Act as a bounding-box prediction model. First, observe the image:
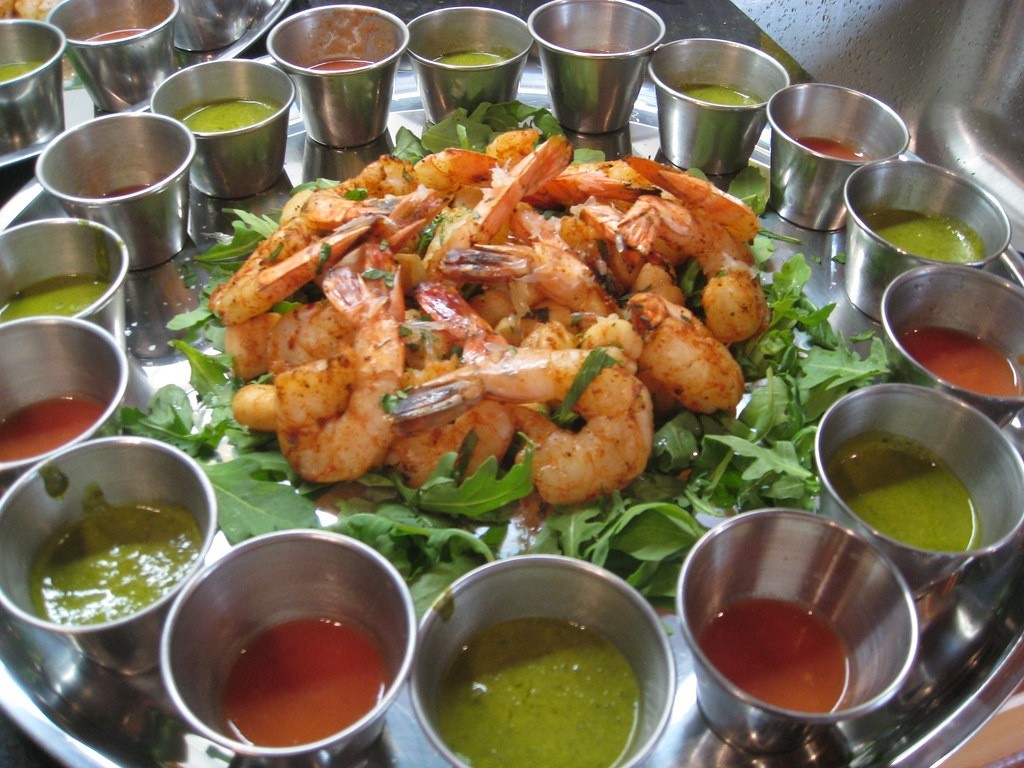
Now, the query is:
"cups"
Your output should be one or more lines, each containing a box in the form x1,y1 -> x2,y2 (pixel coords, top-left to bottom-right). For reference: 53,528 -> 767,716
816,381 -> 1024,599
528,0 -> 666,133
161,530 -> 417,768
409,554 -> 677,768
151,58 -> 298,198
766,82 -> 912,231
266,3 -> 410,148
843,161 -> 1012,321
677,508 -> 918,756
879,264 -> 1024,426
176,0 -> 249,52
406,6 -> 533,124
0,1 -> 217,768
647,38 -> 790,175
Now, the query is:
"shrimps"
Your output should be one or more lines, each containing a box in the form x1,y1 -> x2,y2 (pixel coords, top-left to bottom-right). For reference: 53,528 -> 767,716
202,128 -> 767,509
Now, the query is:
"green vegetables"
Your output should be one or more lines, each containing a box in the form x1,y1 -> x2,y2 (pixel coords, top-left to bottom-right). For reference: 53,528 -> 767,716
118,101 -> 894,636
